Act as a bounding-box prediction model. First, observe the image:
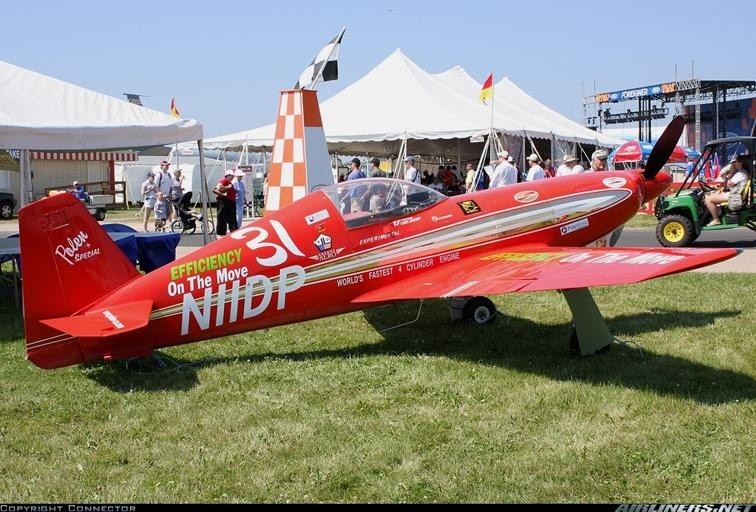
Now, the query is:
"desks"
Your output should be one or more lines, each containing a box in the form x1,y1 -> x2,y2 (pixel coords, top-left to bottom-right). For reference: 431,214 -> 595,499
0,230 -> 181,311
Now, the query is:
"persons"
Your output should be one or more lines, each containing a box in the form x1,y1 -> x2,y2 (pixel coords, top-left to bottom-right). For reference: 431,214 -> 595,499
335,150 -> 608,212
67,181 -> 85,200
705,155 -> 750,226
141,161 -> 268,240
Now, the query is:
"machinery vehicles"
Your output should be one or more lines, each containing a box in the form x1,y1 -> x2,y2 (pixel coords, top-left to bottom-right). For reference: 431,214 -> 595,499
68,189 -> 116,222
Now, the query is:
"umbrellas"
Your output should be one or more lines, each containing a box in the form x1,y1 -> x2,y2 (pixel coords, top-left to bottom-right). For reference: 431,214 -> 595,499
608,140 -> 702,174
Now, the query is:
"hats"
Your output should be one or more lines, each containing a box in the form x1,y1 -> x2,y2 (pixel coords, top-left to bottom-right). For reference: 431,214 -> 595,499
368,157 -> 380,164
161,161 -> 170,166
566,156 -> 577,161
497,151 -> 509,158
234,170 -> 246,176
402,156 -> 415,163
592,150 -> 607,159
225,170 -> 233,176
351,158 -> 360,164
148,172 -> 156,177
527,154 -> 539,161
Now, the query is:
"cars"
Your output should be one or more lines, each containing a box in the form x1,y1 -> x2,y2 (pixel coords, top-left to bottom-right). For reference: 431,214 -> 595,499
0,192 -> 16,218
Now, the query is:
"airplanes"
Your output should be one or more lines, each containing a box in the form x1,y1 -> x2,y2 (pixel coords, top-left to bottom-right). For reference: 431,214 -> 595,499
16,77 -> 747,369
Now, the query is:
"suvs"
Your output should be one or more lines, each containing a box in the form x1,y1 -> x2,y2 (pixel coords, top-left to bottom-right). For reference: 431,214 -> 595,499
654,136 -> 756,245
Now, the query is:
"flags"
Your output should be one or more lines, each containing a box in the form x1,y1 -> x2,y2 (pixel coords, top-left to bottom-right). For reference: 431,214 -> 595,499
171,97 -> 179,117
479,74 -> 493,102
294,27 -> 345,90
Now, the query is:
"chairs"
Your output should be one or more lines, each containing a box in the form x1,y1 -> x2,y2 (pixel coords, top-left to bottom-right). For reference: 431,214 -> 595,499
719,178 -> 751,207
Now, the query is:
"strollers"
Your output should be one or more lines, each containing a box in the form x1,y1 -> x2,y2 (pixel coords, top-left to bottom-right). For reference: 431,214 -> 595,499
145,186 -> 215,237
